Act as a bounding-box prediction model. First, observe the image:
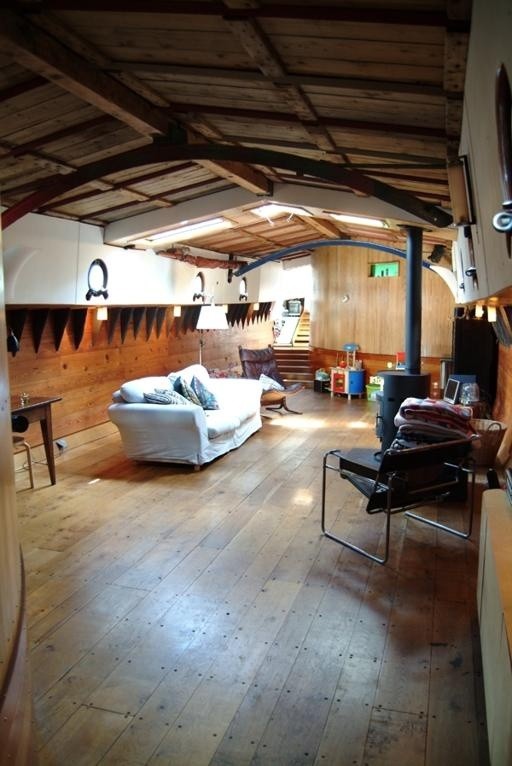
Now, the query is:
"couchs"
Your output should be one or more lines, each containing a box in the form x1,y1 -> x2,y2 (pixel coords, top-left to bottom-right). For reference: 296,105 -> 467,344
107,363 -> 265,472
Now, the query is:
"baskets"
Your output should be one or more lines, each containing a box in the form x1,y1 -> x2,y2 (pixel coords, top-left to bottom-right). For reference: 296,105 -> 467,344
470,418 -> 508,465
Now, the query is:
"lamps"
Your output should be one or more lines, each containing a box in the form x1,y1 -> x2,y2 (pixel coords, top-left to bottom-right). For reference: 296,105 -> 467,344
173,305 -> 181,317
446,154 -> 478,229
487,306 -> 497,323
194,238 -> 459,331
96,306 -> 108,321
474,304 -> 484,317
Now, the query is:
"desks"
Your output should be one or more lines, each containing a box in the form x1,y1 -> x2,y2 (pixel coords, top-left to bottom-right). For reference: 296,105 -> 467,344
11,395 -> 62,486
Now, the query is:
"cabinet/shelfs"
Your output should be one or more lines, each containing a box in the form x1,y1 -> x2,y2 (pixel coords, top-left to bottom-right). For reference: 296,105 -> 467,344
328,366 -> 365,400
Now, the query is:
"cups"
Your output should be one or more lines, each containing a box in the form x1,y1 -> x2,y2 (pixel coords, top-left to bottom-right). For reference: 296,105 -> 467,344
20,392 -> 29,404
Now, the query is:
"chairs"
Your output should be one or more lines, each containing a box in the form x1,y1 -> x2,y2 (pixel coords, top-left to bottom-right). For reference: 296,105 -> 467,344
238,344 -> 305,415
11,415 -> 34,489
321,434 -> 482,564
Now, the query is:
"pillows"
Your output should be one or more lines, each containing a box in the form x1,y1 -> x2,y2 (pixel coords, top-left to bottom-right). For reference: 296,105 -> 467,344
154,388 -> 192,405
190,376 -> 220,410
143,393 -> 179,405
167,364 -> 209,386
173,376 -> 201,405
119,376 -> 174,402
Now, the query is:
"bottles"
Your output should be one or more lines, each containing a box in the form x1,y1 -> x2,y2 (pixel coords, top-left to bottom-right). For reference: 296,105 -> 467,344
432,383 -> 439,398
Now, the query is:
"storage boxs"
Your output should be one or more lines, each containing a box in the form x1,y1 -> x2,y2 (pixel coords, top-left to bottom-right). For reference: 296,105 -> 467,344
314,380 -> 330,393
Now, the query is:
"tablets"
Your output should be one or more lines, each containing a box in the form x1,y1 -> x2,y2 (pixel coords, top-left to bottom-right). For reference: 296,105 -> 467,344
443,378 -> 460,405
